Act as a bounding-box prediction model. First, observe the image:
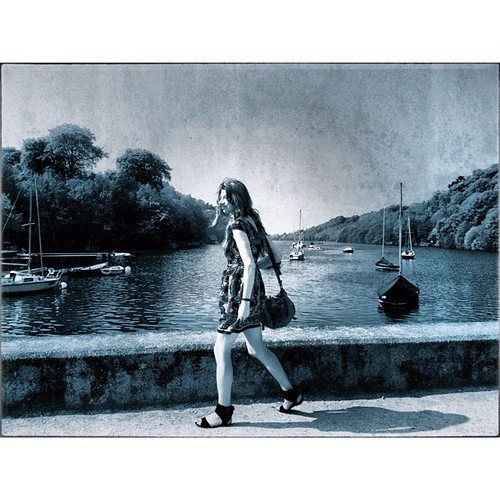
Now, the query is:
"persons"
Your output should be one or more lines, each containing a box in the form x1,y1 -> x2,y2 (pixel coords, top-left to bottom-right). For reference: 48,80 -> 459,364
195,178 -> 304,430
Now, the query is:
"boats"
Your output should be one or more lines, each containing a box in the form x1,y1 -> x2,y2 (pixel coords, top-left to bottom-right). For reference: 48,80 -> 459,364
100,265 -> 124,275
0,149 -> 62,297
290,209 -> 316,261
342,246 -> 354,254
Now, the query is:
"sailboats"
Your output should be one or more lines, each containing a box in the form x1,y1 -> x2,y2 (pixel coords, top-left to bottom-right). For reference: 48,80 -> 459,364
377,182 -> 420,307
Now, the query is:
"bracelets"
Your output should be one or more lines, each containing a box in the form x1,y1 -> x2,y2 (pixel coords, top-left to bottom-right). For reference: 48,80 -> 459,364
242,298 -> 252,301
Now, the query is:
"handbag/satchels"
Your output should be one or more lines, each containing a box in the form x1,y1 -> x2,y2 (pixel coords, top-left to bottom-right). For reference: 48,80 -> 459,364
259,287 -> 296,331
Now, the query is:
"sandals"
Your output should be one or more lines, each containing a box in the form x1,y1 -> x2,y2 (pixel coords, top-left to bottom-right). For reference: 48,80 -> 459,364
276,388 -> 304,413
194,402 -> 235,428
